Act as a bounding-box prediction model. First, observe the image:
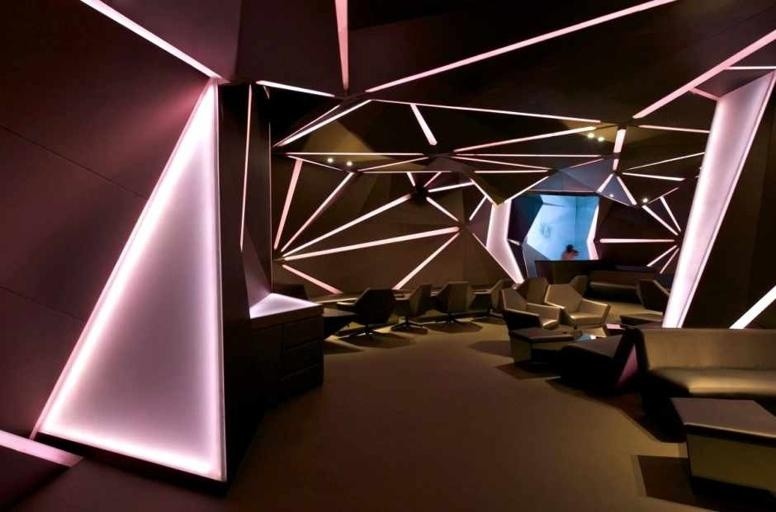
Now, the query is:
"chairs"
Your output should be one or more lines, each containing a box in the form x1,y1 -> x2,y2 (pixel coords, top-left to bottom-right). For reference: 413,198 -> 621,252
557,321 -> 662,393
272,276 -> 610,341
636,278 -> 670,312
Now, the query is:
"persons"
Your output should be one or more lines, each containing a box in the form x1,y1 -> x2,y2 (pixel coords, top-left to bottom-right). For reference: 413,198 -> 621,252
562,245 -> 578,260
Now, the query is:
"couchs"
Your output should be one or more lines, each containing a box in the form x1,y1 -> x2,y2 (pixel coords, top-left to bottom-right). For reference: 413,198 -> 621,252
634,327 -> 775,442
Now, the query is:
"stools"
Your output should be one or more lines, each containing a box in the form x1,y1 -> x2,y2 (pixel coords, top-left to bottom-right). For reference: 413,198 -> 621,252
620,313 -> 665,328
669,396 -> 775,510
